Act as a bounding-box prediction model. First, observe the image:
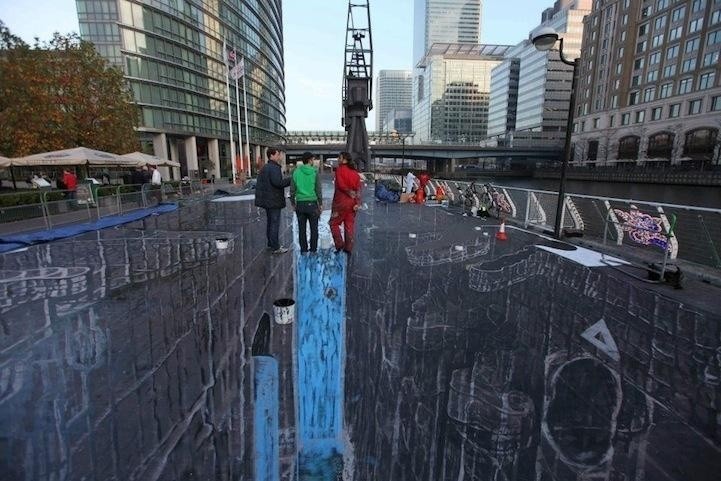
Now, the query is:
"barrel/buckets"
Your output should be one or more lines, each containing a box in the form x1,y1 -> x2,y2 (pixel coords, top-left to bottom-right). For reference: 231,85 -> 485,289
273,296 -> 296,325
215,237 -> 230,251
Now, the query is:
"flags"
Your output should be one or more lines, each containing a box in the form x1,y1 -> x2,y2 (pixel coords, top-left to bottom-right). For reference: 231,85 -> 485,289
228,49 -> 236,62
230,58 -> 244,80
222,42 -> 229,65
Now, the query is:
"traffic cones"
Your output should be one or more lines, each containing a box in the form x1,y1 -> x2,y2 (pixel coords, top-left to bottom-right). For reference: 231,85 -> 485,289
497,218 -> 509,241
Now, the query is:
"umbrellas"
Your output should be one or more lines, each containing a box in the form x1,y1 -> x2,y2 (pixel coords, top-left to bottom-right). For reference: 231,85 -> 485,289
11,147 -> 140,166
0,157 -> 28,167
121,151 -> 180,167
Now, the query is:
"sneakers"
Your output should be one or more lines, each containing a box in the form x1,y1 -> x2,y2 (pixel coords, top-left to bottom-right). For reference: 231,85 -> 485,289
271,245 -> 287,255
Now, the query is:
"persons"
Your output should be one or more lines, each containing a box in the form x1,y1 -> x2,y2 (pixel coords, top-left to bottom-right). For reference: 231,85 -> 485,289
290,152 -> 323,256
420,170 -> 431,189
328,151 -> 361,254
406,169 -> 416,193
254,147 -> 292,254
26,166 -> 162,205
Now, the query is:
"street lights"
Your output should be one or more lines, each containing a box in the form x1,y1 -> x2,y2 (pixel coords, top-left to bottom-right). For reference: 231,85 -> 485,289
389,129 -> 406,193
526,20 -> 583,241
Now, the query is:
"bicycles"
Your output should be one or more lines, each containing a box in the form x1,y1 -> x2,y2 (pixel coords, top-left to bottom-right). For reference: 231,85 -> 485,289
458,182 -> 500,219
